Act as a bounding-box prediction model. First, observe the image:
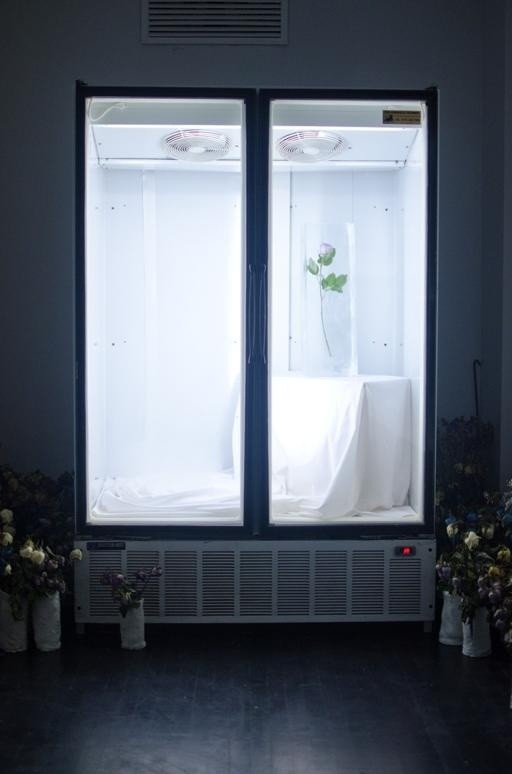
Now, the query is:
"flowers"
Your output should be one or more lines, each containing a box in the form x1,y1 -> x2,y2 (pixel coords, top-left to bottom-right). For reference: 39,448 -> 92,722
0,464 -> 83,621
306,244 -> 348,357
435,415 -> 512,648
99,564 -> 164,618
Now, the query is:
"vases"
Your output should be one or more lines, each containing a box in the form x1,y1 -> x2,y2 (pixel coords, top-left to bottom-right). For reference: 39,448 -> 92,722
0,589 -> 29,653
31,591 -> 62,652
119,597 -> 147,651
439,591 -> 491,658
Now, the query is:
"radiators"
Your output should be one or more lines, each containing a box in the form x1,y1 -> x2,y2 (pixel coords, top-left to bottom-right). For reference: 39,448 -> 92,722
73,540 -> 437,634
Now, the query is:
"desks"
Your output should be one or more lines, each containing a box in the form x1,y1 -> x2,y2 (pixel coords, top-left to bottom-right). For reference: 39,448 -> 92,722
233,373 -> 411,518
